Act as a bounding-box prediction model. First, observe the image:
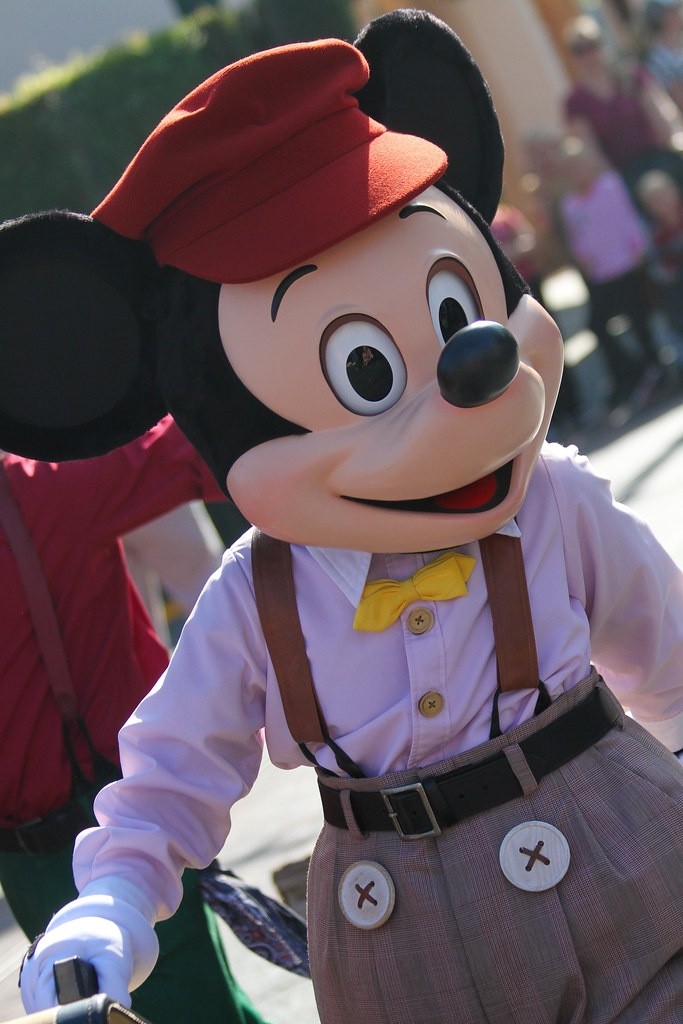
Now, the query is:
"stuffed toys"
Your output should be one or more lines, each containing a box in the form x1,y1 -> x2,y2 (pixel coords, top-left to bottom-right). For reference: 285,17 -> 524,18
0,9 -> 683,1024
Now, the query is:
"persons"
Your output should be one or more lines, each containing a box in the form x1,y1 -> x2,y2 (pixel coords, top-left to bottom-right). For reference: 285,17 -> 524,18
0,404 -> 285,1024
476,0 -> 683,440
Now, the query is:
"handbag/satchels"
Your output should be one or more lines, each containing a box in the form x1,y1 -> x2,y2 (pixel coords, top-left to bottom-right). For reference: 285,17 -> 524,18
552,171 -> 650,287
